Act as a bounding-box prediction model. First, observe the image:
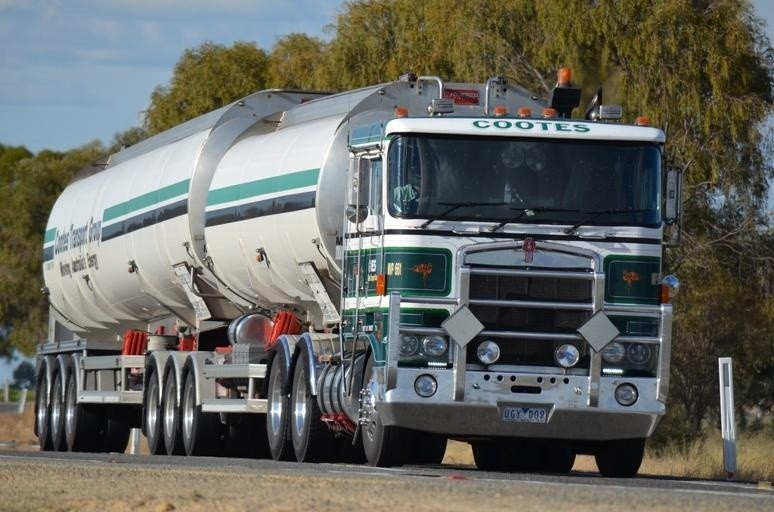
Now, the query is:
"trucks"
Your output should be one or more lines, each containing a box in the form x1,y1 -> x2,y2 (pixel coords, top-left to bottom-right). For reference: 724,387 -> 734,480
31,61 -> 687,477
